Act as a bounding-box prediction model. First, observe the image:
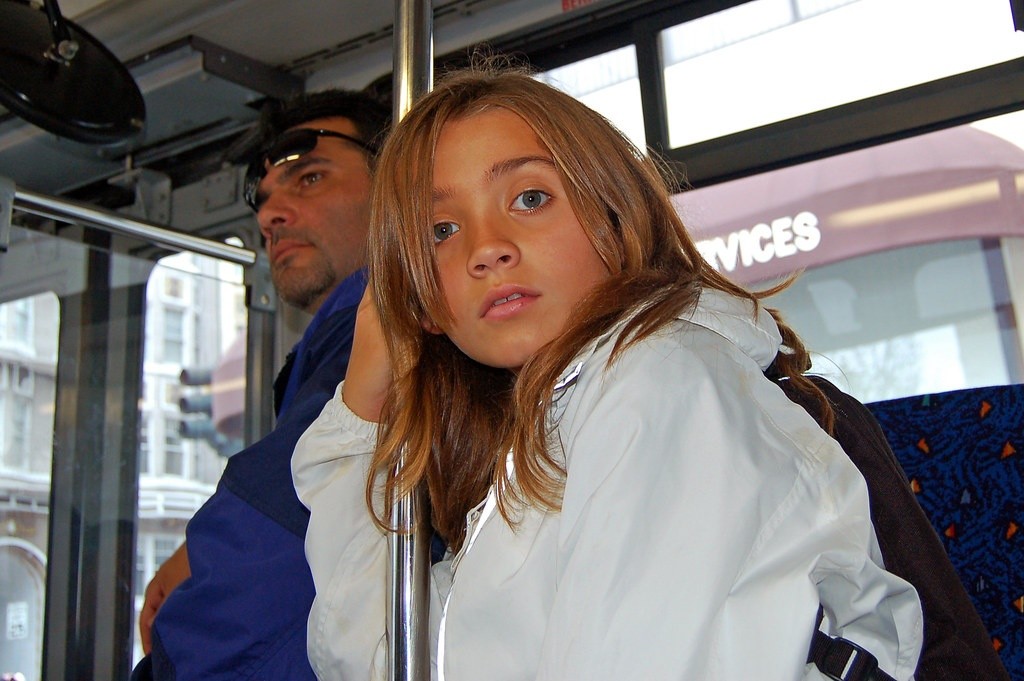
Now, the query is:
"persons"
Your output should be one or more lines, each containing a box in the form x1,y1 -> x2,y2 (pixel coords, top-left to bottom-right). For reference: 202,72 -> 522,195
133,69 -> 923,681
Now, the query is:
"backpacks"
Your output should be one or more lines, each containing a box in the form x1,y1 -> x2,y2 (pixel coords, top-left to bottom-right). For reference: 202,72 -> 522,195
764,349 -> 1011,681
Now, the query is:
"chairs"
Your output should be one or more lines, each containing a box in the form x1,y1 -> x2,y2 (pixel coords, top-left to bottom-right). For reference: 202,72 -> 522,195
859,386 -> 1024,680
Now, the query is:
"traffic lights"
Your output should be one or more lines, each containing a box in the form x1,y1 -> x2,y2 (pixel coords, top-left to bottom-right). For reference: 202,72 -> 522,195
179,365 -> 228,451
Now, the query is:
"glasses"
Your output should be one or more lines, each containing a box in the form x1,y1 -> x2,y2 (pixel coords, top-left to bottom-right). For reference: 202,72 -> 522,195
243,128 -> 378,213
